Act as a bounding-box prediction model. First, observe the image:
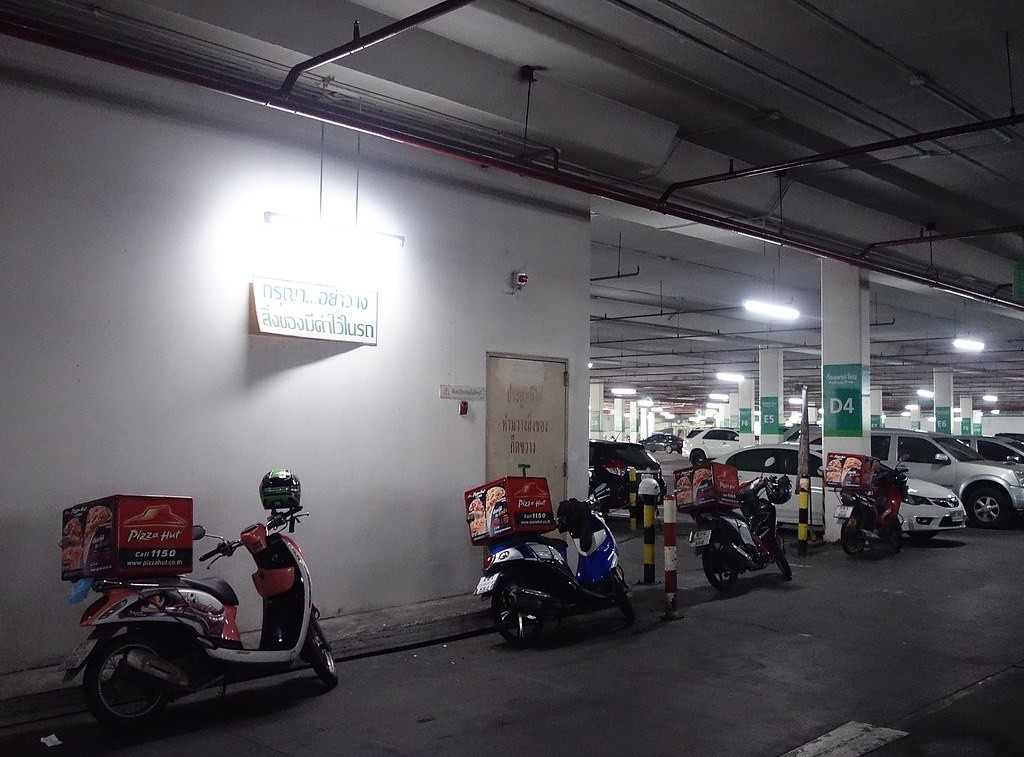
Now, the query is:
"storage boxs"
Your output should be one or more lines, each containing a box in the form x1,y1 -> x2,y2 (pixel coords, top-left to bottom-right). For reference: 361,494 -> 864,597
464,475 -> 558,546
673,461 -> 742,515
62,496 -> 194,581
825,452 -> 881,492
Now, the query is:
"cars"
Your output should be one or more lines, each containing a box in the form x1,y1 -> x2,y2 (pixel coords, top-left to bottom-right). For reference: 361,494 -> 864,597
709,442 -> 969,545
639,434 -> 683,454
683,427 -> 758,467
589,439 -> 668,517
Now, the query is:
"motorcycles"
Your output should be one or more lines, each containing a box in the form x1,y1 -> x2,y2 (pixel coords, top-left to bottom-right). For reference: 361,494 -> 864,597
55,500 -> 341,728
678,468 -> 792,593
470,485 -> 638,647
816,452 -> 910,560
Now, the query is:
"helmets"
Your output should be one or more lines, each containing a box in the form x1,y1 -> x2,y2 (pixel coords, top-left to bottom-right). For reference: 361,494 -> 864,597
767,476 -> 792,505
260,469 -> 301,509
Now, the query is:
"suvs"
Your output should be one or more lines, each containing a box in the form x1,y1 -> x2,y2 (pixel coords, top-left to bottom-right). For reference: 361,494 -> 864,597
796,429 -> 1024,527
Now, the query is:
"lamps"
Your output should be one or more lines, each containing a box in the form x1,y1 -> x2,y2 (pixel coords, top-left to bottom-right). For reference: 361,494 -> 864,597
743,243 -> 801,321
588,360 -> 1003,422
953,299 -> 985,350
261,93 -> 407,246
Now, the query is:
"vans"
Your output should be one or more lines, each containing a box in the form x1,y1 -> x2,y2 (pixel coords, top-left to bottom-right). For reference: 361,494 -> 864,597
995,432 -> 1024,442
955,434 -> 1024,470
